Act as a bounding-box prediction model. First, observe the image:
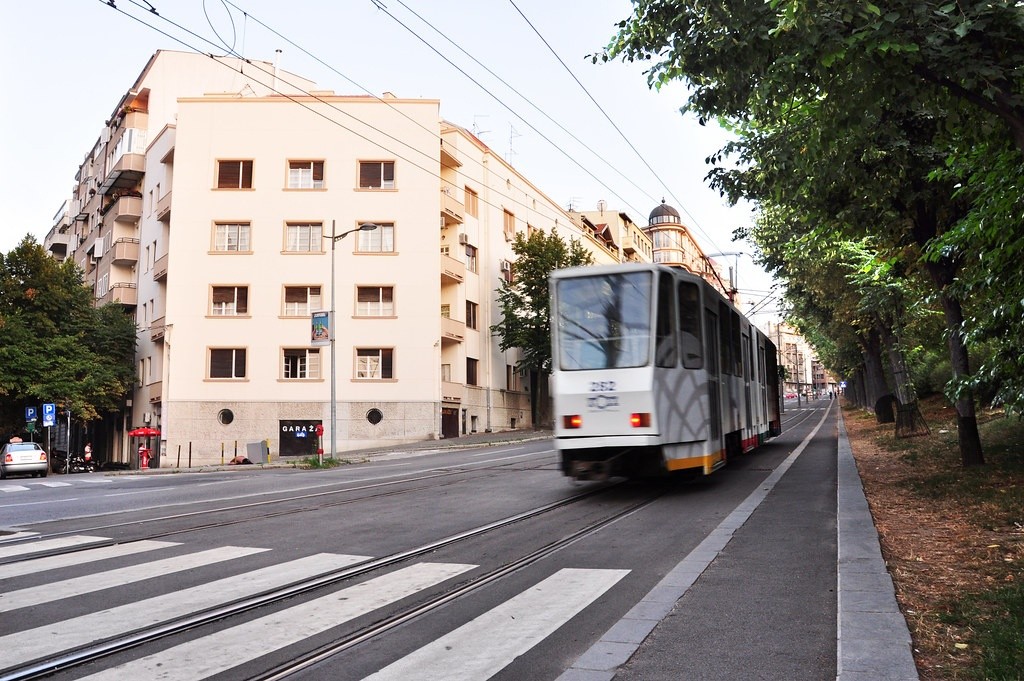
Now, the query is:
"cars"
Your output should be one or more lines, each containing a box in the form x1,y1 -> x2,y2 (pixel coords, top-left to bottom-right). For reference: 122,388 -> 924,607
0,437 -> 48,480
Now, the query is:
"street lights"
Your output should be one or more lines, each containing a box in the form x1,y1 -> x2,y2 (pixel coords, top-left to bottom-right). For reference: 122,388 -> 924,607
330,219 -> 376,460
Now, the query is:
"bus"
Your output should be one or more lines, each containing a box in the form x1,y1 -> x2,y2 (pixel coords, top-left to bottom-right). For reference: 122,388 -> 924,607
547,262 -> 787,494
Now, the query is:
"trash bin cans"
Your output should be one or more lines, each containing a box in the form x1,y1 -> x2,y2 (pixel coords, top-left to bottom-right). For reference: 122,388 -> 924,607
247,441 -> 267,463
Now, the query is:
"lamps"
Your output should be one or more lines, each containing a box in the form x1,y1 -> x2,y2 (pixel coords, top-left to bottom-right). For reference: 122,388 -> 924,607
126,400 -> 133,411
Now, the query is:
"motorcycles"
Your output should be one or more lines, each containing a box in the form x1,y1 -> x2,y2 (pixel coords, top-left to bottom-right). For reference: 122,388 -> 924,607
52,451 -> 95,475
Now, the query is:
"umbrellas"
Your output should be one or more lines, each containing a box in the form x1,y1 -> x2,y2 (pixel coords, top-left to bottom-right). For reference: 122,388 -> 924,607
129,427 -> 161,449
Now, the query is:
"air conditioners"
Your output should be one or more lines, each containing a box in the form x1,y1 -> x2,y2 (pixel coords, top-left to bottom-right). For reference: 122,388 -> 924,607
501,261 -> 510,272
441,217 -> 446,228
90,255 -> 96,264
143,412 -> 150,423
460,233 -> 469,244
505,232 -> 514,242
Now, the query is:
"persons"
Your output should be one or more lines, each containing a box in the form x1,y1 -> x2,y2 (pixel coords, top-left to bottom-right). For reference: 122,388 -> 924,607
138,443 -> 145,453
84,442 -> 93,473
